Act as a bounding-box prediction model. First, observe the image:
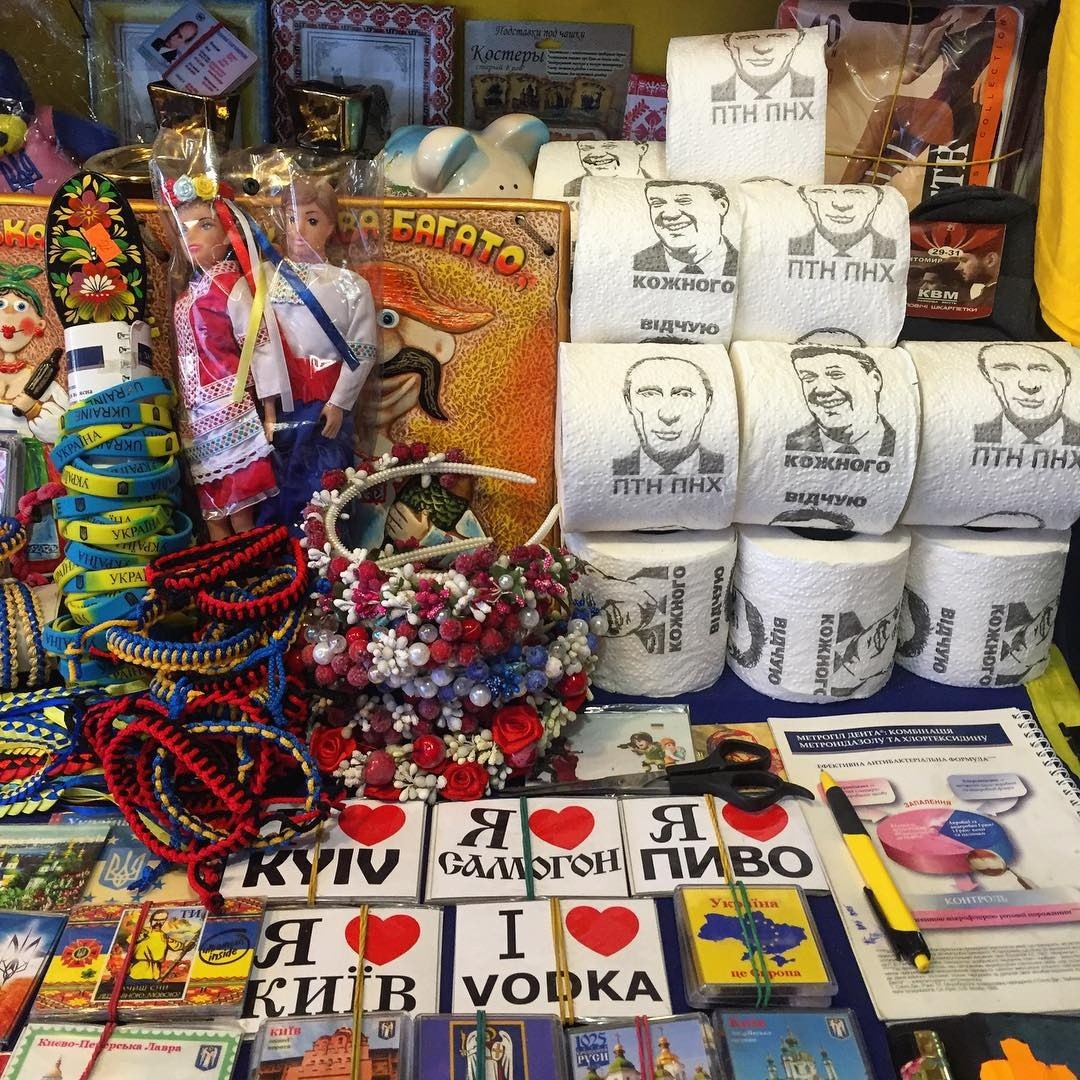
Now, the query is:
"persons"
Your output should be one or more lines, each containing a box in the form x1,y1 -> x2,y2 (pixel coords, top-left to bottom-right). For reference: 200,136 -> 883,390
920,261 -> 973,306
153,19 -> 198,62
156,178 -> 291,544
956,249 -> 1002,309
265,182 -> 377,544
838,6 -> 997,185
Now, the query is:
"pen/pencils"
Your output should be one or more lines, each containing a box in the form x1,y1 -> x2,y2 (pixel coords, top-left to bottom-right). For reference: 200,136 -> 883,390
820,768 -> 931,973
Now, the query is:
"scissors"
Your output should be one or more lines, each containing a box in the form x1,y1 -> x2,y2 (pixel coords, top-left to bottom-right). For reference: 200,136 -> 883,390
501,739 -> 815,813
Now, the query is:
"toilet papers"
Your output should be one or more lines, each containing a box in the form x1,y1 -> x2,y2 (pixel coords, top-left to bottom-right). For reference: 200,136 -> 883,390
529,135 -> 670,257
718,521 -> 914,707
558,524 -> 745,706
897,332 -> 1080,542
660,23 -> 833,198
567,171 -> 745,352
738,176 -> 914,348
725,343 -> 922,538
905,521 -> 1070,692
550,336 -> 739,548
